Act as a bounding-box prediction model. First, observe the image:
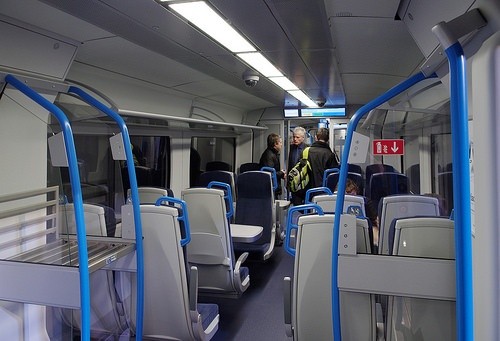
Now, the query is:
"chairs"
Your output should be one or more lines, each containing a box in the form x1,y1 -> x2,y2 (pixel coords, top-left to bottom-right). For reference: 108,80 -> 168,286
52,162 -> 456,341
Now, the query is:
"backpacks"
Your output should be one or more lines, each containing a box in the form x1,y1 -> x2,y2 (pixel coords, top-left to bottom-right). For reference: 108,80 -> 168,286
287,147 -> 312,193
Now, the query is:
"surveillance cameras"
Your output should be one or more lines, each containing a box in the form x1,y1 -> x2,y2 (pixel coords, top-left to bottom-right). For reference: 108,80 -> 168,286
245,75 -> 259,88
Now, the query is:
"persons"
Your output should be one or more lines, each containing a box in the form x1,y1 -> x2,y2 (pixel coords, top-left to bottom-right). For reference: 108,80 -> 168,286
299,128 -> 340,213
287,126 -> 311,209
257,132 -> 287,246
333,177 -> 378,227
